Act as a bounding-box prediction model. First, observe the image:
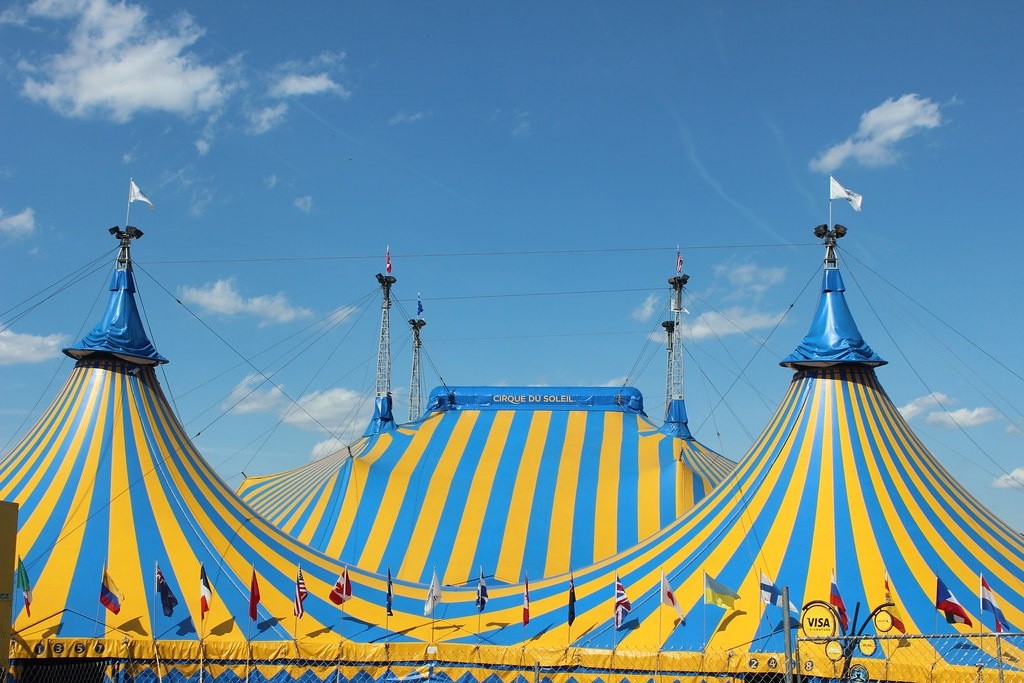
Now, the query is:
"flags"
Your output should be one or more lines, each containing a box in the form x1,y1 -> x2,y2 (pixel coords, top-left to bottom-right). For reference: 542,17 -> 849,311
676,249 -> 684,274
386,245 -> 392,273
17,555 -> 33,618
830,176 -> 863,212
99,562 -> 1015,635
416,292 -> 424,316
129,179 -> 156,212
670,298 -> 691,315
